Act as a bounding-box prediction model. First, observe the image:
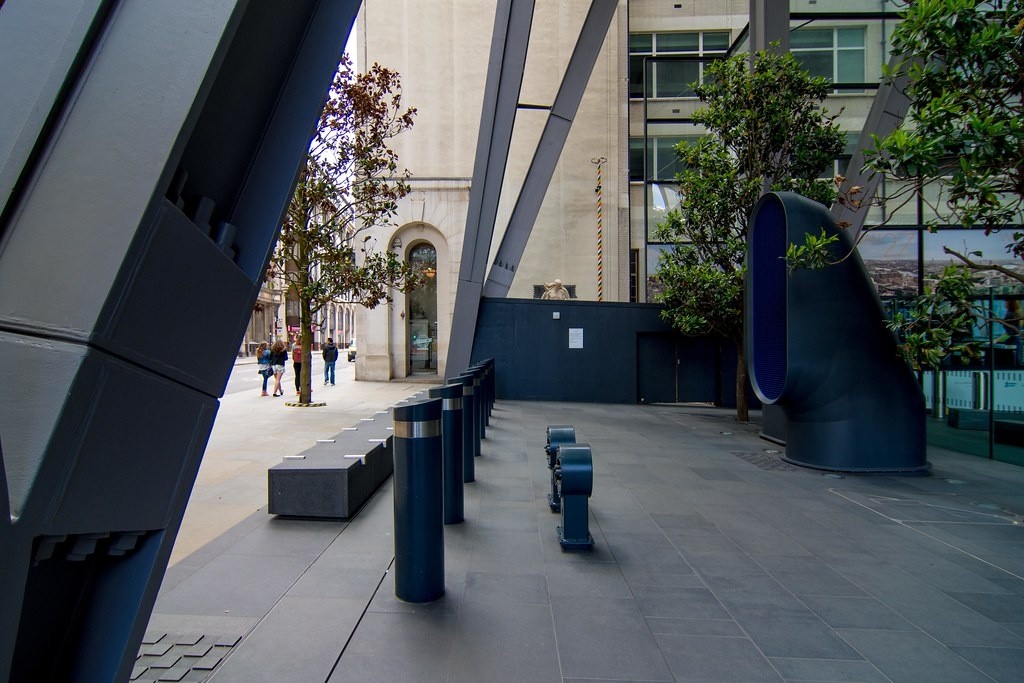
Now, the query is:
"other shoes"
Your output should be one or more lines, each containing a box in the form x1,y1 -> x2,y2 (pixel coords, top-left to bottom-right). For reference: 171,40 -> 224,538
281,390 -> 284,395
324,381 -> 329,385
273,394 -> 280,397
331,383 -> 335,386
297,391 -> 300,395
311,389 -> 313,392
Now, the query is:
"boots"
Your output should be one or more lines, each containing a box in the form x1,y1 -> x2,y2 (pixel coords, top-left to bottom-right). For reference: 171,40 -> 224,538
261,391 -> 270,396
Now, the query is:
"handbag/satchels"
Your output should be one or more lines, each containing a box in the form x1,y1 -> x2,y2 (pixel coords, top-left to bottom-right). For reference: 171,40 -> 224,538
266,367 -> 274,377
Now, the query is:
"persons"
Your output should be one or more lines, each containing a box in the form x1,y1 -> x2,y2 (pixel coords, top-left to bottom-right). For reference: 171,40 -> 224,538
292,333 -> 313,395
256,339 -> 289,397
322,337 -> 338,386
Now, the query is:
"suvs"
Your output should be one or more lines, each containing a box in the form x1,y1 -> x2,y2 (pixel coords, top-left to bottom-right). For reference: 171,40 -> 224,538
346,339 -> 356,362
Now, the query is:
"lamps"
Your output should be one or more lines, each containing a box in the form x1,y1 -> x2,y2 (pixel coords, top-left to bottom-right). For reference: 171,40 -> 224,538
400,311 -> 405,320
